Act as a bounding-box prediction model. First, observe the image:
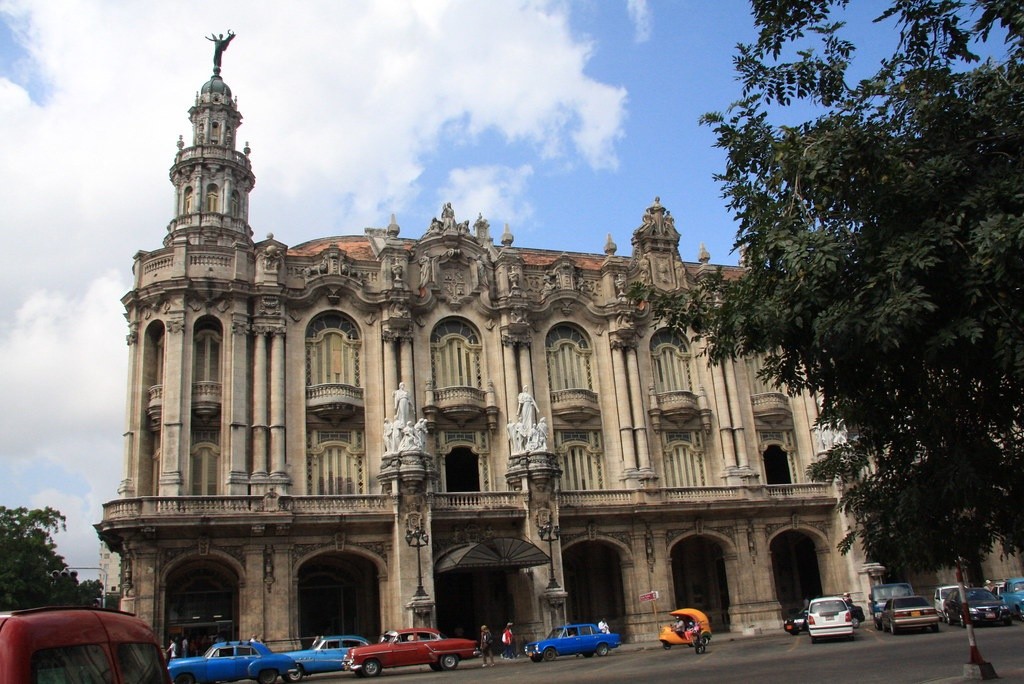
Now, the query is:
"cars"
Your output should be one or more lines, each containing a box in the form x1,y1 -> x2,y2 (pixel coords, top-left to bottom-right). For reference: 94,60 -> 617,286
274,634 -> 374,682
165,640 -> 298,684
523,623 -> 622,663
340,627 -> 483,678
784,600 -> 865,635
0,604 -> 172,684
867,578 -> 1024,635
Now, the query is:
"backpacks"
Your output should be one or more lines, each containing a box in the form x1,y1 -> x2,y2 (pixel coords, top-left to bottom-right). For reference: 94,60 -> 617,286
484,631 -> 493,645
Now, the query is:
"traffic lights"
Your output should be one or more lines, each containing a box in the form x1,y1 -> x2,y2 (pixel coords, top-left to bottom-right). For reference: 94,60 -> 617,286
51,570 -> 78,578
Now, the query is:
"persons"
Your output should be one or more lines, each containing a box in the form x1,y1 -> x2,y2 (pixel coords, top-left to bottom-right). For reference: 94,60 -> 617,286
164,638 -> 178,662
598,617 -> 612,652
542,267 -> 557,288
205,28 -> 235,67
719,593 -> 730,624
382,381 -> 429,455
984,580 -> 995,593
480,625 -> 495,668
642,196 -> 678,234
505,383 -> 550,456
798,591 -> 812,614
566,629 -> 576,638
180,633 -> 210,658
670,614 -> 704,641
427,201 -> 471,235
576,271 -> 586,291
475,253 -> 489,288
418,250 -> 431,289
500,622 -> 520,661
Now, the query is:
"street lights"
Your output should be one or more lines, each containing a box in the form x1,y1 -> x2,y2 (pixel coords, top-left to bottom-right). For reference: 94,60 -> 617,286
537,523 -> 563,589
405,525 -> 430,596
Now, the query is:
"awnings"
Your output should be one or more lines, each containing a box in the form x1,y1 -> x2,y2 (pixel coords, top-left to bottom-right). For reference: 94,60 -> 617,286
433,536 -> 552,574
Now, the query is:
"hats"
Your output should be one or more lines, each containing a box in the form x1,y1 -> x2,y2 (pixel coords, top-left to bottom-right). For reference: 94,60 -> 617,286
481,625 -> 488,630
507,622 -> 513,626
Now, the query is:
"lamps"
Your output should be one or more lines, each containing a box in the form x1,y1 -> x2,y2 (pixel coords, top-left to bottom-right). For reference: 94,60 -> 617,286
524,568 -> 534,582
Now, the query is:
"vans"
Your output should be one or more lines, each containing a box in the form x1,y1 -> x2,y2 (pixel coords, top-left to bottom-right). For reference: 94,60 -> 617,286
804,596 -> 853,644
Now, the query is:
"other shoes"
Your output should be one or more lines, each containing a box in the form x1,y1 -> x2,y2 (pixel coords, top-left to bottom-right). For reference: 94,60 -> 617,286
514,654 -> 519,658
482,663 -> 495,667
505,657 -> 514,660
500,653 -> 504,659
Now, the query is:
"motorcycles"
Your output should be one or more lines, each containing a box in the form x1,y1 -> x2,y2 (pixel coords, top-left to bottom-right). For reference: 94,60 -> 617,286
659,606 -> 713,649
683,620 -> 712,654
